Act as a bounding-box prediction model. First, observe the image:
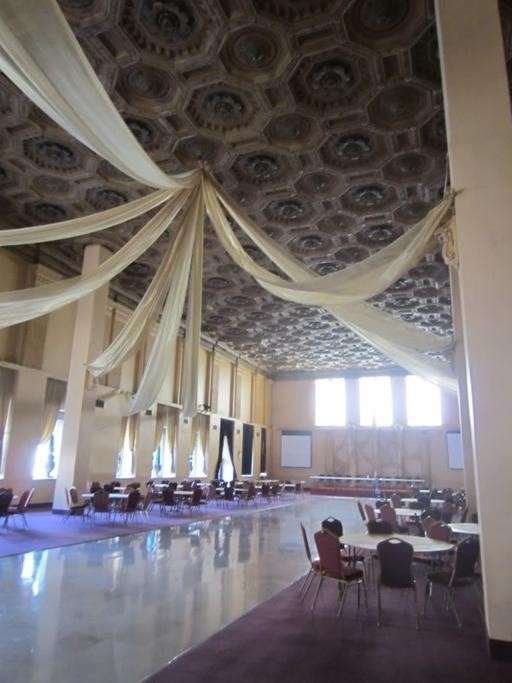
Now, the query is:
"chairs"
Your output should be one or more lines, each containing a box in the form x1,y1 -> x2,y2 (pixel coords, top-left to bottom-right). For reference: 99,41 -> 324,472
309,474 -> 423,488
298,488 -> 477,630
0,479 -> 301,531
